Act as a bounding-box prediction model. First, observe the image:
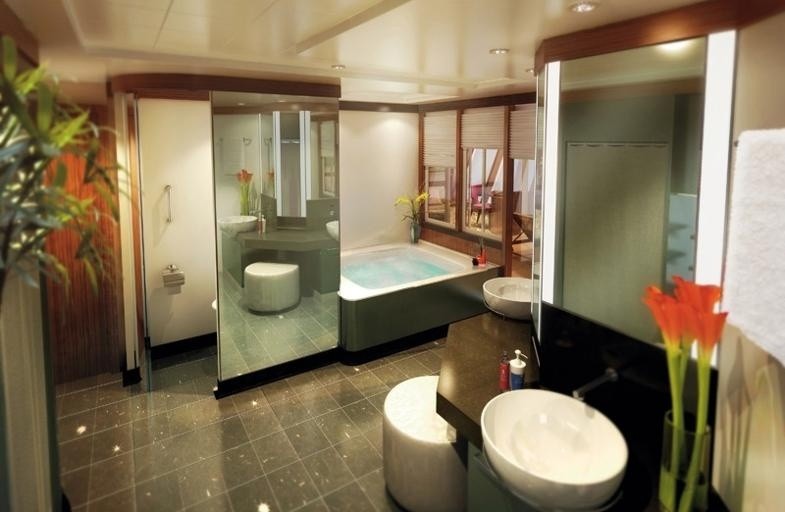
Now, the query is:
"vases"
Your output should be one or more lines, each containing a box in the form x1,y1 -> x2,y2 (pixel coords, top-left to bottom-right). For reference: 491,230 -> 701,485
658,409 -> 711,512
410,222 -> 418,244
238,200 -> 250,216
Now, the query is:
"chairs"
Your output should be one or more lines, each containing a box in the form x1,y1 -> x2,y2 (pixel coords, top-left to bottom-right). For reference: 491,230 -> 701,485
471,184 -> 492,225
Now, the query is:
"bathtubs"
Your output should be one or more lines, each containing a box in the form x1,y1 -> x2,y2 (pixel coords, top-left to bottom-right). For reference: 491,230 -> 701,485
338,239 -> 500,351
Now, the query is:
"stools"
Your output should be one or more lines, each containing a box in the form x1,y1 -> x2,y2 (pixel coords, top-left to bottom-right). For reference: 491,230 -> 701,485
383,375 -> 468,512
244,260 -> 300,315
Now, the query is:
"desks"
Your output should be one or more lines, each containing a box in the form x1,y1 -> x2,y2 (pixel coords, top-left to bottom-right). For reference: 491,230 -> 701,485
491,191 -> 522,235
238,229 -> 320,262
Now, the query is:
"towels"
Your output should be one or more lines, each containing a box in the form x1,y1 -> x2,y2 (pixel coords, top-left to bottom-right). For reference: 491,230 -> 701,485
722,128 -> 785,367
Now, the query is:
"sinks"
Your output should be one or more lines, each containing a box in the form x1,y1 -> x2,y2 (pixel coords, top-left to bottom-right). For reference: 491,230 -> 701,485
218,216 -> 258,234
483,277 -> 531,320
326,220 -> 338,242
481,388 -> 629,512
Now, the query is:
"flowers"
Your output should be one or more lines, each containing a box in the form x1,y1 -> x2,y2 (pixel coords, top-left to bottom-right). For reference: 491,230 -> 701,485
236,169 -> 253,216
394,192 -> 430,224
641,275 -> 729,512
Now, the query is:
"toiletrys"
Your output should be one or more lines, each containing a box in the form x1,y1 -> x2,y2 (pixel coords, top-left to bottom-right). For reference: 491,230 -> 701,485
509,350 -> 528,389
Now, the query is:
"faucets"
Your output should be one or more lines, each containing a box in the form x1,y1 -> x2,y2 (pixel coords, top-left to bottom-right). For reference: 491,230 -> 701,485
250,209 -> 266,216
573,367 -> 617,401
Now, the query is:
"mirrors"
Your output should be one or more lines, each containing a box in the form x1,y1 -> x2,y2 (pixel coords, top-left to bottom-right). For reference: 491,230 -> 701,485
210,90 -> 340,381
551,35 -> 708,359
